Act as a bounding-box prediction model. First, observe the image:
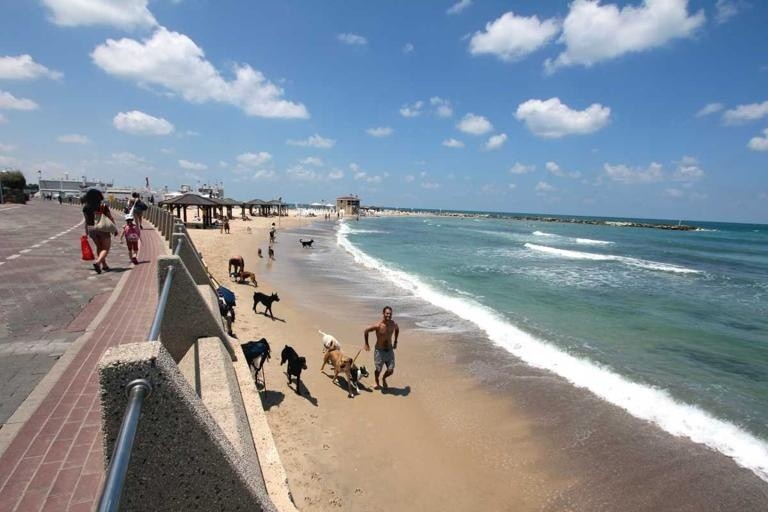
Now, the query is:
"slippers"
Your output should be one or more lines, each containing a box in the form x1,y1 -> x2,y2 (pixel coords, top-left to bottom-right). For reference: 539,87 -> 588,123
93,262 -> 109,274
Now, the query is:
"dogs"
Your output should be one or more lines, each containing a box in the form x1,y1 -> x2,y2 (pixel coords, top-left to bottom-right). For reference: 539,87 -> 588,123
268,245 -> 274,258
280,345 -> 308,394
252,291 -> 280,321
234,270 -> 257,287
350,365 -> 370,395
318,329 -> 341,354
320,350 -> 354,397
299,238 -> 315,249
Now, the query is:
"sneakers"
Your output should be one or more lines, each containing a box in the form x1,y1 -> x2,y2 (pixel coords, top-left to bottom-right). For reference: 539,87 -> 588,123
132,256 -> 138,265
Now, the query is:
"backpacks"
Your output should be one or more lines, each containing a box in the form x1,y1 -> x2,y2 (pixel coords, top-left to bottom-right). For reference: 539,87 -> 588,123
124,224 -> 140,242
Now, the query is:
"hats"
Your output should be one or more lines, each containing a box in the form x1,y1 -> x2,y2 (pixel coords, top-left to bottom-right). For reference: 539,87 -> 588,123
125,213 -> 134,221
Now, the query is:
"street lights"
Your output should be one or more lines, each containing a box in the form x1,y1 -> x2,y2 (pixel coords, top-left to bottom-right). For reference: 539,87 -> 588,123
278,196 -> 283,227
321,199 -> 324,213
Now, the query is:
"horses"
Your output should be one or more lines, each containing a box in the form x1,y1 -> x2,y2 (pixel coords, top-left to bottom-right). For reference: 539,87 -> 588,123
224,220 -> 230,234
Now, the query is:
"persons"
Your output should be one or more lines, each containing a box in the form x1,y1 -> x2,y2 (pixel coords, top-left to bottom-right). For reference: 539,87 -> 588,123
128,192 -> 136,214
58,194 -> 64,206
132,193 -> 143,229
363,306 -> 399,391
120,214 -> 140,265
69,195 -> 73,204
83,189 -> 118,274
219,216 -> 230,233
269,222 -> 276,243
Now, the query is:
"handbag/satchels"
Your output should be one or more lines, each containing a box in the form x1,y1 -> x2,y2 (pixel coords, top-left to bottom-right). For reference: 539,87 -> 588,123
135,199 -> 148,210
93,203 -> 117,233
80,233 -> 96,261
